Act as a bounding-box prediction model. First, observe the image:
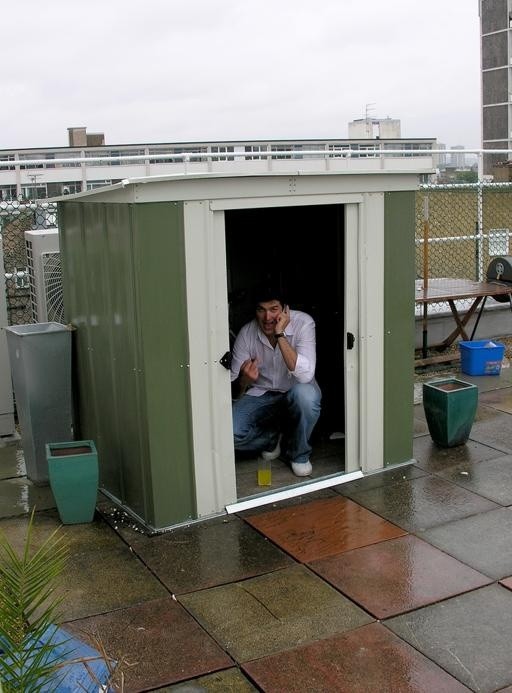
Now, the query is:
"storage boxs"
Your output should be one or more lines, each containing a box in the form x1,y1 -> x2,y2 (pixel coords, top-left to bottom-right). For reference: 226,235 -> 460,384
459,340 -> 505,376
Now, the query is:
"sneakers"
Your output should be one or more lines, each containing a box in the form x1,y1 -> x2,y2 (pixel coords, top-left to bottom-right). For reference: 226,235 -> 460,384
291,460 -> 313,477
262,434 -> 281,460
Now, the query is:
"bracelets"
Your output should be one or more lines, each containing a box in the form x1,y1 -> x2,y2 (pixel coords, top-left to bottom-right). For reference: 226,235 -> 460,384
273,332 -> 285,339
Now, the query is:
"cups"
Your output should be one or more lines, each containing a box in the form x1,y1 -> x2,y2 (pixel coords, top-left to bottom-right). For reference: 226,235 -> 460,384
256,456 -> 272,486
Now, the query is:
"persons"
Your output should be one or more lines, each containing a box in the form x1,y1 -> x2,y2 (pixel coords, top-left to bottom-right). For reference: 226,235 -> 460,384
229,291 -> 322,476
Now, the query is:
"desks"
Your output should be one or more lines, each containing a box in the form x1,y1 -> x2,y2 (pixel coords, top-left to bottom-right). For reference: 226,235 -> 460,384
415,278 -> 512,353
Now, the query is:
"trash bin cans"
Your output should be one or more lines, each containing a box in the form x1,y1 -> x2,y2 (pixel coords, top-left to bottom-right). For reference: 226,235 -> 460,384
2,322 -> 77,487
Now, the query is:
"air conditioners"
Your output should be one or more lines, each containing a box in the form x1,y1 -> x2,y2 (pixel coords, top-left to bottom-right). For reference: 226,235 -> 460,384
24,228 -> 64,324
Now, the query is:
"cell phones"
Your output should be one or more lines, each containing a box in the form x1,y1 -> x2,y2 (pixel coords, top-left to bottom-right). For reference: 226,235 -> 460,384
281,303 -> 287,313
